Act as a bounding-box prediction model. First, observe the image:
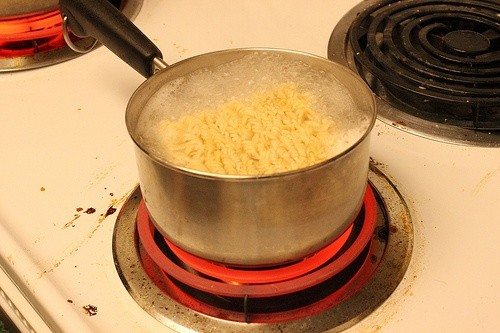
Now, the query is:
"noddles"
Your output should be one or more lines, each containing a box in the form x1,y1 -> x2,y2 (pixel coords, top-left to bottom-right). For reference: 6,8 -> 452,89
151,82 -> 346,177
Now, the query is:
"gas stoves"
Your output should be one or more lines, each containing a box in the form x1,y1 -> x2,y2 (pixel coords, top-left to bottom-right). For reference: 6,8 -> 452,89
1,0 -> 500,333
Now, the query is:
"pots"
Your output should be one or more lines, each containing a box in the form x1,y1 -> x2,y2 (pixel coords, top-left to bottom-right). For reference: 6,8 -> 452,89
59,0 -> 382,263
